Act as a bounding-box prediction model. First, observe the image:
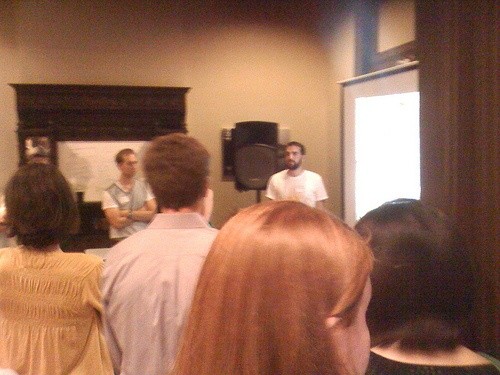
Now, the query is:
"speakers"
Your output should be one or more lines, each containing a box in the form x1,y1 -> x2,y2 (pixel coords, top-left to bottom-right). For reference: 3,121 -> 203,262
232,121 -> 278,193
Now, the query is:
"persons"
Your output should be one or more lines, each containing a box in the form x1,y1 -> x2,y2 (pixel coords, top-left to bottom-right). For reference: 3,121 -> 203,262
355,199 -> 499,375
101,149 -> 157,239
173,200 -> 376,375
265,141 -> 328,210
0,164 -> 114,375
101,133 -> 225,375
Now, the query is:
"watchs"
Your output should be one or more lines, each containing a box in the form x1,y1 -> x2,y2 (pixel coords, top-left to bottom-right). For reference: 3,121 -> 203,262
128,210 -> 132,218
